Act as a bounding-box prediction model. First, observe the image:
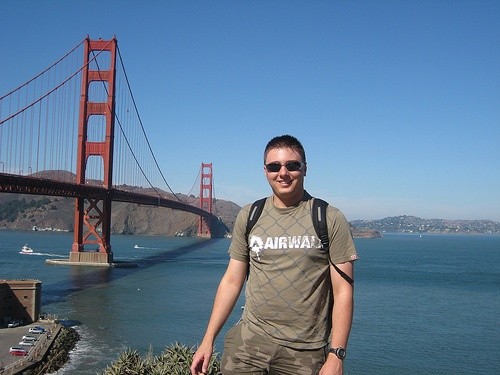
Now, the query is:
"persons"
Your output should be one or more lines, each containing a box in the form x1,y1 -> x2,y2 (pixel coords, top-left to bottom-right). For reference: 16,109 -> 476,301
189,135 -> 359,375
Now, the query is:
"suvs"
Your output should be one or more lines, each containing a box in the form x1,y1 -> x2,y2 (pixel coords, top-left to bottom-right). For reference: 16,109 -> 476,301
29,328 -> 43,334
22,335 -> 36,341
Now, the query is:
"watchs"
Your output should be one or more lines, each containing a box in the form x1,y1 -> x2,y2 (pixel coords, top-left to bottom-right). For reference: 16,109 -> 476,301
329,347 -> 346,360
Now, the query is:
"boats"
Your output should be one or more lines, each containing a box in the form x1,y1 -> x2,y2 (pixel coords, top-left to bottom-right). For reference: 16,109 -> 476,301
21,247 -> 34,253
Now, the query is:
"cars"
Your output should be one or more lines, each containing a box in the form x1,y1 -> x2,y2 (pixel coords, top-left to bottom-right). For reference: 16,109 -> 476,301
35,326 -> 45,331
19,341 -> 34,346
12,351 -> 28,356
9,347 -> 24,353
8,320 -> 23,328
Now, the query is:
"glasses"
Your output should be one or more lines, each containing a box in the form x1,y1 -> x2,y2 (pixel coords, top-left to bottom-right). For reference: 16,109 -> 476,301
265,160 -> 306,172
